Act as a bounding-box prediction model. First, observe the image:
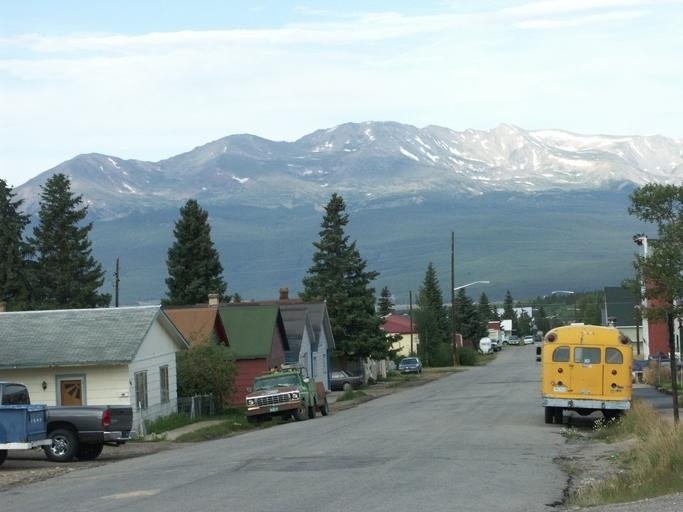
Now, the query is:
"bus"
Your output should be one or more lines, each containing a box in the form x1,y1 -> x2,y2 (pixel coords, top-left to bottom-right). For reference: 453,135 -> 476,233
535,323 -> 634,426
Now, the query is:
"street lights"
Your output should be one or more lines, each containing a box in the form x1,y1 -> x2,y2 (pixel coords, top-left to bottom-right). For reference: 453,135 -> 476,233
451,279 -> 490,367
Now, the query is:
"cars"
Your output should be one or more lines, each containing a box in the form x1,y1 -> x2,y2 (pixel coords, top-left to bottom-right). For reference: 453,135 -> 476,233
398,357 -> 424,375
479,333 -> 542,356
329,369 -> 364,392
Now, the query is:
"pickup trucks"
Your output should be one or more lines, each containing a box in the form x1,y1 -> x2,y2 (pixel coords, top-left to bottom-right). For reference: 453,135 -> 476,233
0,380 -> 132,464
0,403 -> 54,466
244,366 -> 329,427
